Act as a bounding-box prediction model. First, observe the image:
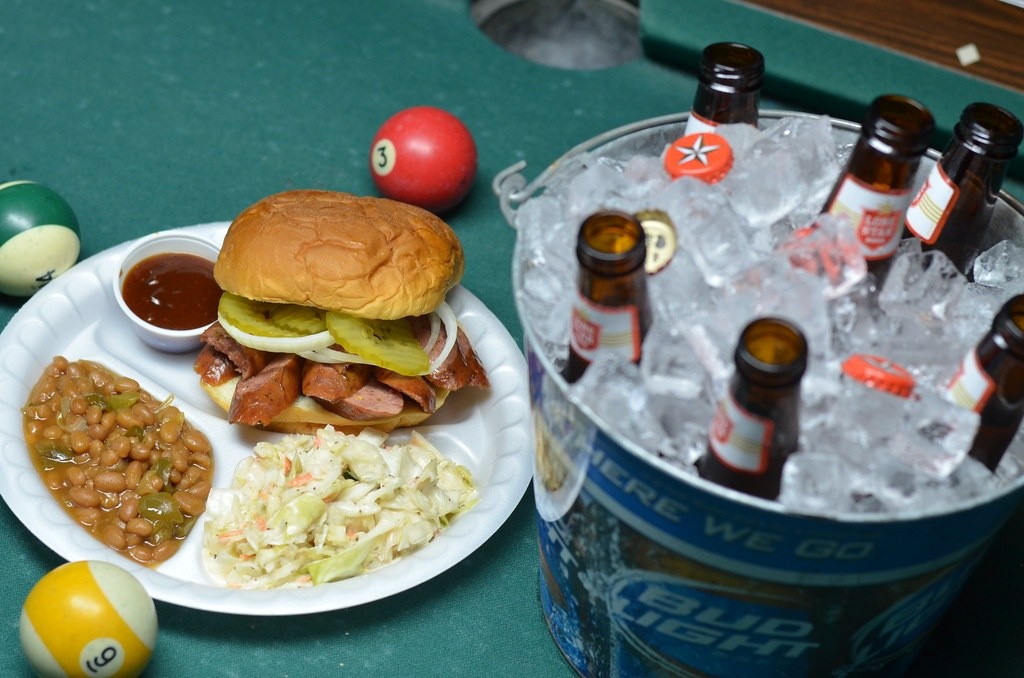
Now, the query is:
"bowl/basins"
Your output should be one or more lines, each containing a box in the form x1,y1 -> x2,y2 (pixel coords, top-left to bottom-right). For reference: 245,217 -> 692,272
112,229 -> 222,354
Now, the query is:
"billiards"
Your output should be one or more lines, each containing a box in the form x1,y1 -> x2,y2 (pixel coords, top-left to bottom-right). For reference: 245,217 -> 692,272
19,559 -> 159,677
0,179 -> 80,298
371,106 -> 477,214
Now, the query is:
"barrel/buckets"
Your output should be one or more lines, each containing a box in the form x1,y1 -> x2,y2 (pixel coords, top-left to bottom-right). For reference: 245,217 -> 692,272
512,112 -> 1021,676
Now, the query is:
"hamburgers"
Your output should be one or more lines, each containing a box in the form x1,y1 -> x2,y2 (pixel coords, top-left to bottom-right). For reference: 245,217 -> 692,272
194,191 -> 490,435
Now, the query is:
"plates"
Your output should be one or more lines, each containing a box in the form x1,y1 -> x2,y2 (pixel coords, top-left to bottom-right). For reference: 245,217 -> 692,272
0,220 -> 533,615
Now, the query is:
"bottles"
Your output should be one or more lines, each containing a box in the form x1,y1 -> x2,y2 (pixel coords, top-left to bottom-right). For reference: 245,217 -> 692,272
565,207 -> 657,390
810,93 -> 935,292
944,295 -> 1023,472
898,101 -> 1022,286
682,42 -> 767,138
693,315 -> 809,501
